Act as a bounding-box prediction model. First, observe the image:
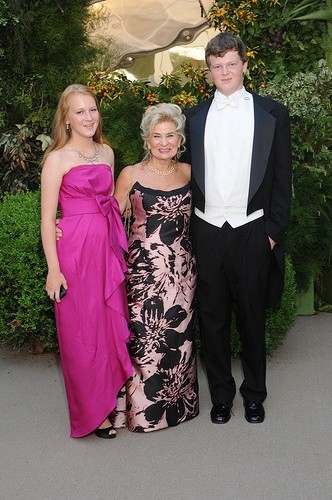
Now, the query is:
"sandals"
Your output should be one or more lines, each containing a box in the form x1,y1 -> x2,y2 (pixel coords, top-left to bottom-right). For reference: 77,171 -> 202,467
96,422 -> 117,439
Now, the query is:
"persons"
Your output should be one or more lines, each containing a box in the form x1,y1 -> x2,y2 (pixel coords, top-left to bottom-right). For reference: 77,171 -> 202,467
178,32 -> 292,423
55,103 -> 198,434
39,84 -> 130,441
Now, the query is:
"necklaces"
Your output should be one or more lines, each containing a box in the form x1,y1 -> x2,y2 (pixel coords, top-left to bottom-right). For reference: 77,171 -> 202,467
146,158 -> 177,175
68,139 -> 102,163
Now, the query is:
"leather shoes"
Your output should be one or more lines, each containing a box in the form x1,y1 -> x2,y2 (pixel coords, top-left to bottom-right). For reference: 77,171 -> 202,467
210,403 -> 233,424
242,398 -> 265,423
